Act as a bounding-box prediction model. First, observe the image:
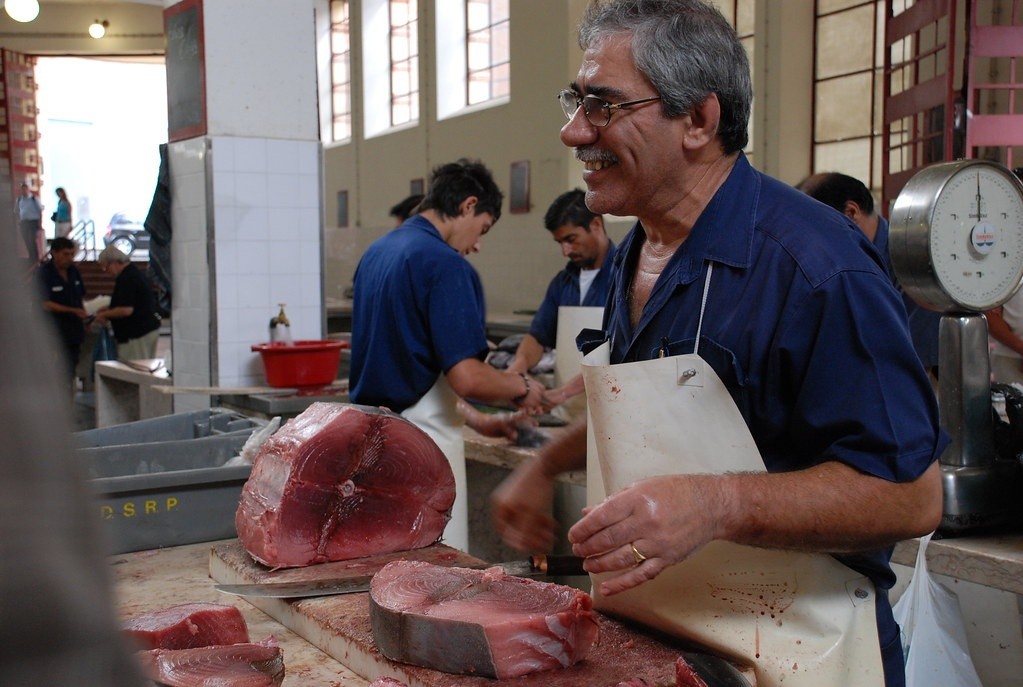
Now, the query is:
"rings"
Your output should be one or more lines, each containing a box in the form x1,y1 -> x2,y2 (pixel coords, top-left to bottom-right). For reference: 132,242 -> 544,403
630,543 -> 646,567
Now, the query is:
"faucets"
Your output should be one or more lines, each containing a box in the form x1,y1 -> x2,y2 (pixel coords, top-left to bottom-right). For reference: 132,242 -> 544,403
268,302 -> 291,329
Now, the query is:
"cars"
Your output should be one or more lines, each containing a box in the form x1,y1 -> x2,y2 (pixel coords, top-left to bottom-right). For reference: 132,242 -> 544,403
103,210 -> 150,258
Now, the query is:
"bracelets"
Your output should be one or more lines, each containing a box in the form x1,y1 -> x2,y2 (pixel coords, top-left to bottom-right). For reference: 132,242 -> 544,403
511,372 -> 530,403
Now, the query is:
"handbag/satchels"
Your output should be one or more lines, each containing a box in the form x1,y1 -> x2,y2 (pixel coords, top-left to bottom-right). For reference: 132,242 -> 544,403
890,529 -> 982,687
91,325 -> 117,379
51,213 -> 57,222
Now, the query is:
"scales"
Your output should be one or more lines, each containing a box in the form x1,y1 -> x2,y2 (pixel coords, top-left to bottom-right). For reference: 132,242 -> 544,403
888,159 -> 1023,538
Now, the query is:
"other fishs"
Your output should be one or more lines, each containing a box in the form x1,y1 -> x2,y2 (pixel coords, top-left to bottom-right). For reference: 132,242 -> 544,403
121,399 -> 598,687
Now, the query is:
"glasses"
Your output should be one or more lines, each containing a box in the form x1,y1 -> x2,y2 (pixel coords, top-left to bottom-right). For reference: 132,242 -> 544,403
558,89 -> 662,127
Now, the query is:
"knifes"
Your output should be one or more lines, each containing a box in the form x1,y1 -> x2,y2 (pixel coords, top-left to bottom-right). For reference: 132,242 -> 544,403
213,555 -> 585,597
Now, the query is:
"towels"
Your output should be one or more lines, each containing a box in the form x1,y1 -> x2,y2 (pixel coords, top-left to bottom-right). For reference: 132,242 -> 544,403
143,143 -> 173,321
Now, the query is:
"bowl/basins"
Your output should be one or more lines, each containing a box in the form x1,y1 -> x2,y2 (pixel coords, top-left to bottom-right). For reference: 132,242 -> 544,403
250,341 -> 348,386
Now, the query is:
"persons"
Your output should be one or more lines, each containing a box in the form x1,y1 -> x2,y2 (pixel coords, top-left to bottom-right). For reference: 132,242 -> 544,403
506,188 -> 618,424
491,0 -> 954,687
347,157 -> 545,554
93,246 -> 162,396
31,237 -> 87,380
55,188 -> 73,239
987,168 -> 1023,386
15,184 -> 42,260
389,194 -> 425,229
795,172 -> 943,382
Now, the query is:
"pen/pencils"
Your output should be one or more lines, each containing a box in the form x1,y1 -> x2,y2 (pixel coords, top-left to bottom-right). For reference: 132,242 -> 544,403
658,346 -> 669,358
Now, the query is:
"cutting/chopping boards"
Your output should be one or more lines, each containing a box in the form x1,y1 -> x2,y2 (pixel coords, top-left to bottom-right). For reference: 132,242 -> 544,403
208,539 -> 684,685
